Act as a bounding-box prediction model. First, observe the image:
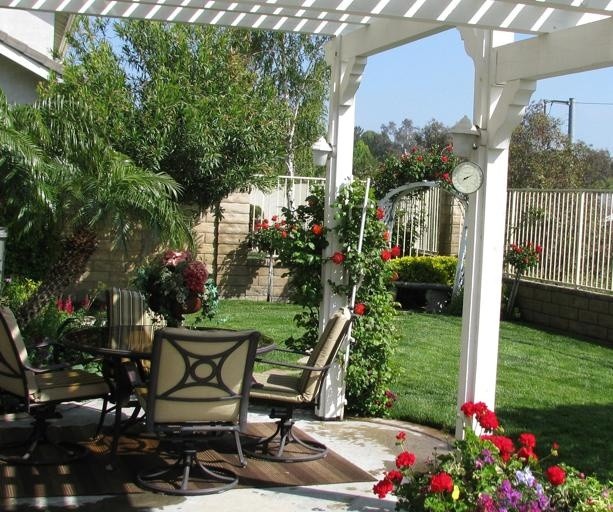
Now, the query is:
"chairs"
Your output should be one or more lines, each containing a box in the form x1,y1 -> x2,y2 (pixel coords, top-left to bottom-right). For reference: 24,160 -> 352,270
0,305 -> 111,466
91,286 -> 152,444
233,306 -> 353,462
135,328 -> 260,495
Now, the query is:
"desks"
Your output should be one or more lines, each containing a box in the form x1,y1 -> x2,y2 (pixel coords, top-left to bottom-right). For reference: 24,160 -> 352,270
65,323 -> 276,470
